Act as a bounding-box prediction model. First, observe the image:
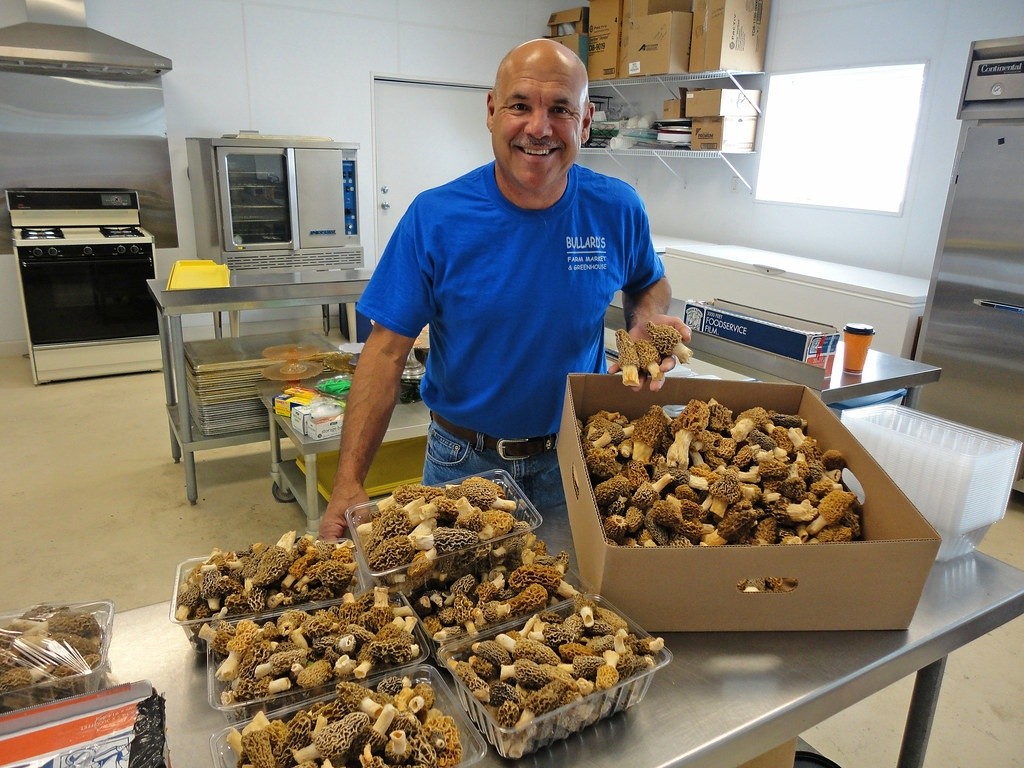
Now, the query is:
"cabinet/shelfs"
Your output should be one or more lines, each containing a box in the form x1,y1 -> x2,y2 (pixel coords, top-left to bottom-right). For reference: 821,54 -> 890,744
144,268 -> 375,506
256,372 -> 431,538
577,70 -> 766,191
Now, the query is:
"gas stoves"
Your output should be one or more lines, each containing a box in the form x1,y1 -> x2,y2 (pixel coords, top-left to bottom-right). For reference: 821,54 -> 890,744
3,188 -> 155,246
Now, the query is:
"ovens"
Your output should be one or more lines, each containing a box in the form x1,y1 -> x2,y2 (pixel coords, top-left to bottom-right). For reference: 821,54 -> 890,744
14,243 -> 165,386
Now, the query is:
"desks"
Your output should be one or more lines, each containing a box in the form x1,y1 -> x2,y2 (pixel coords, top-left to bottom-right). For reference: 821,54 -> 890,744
604,293 -> 942,410
98,505 -> 1024,768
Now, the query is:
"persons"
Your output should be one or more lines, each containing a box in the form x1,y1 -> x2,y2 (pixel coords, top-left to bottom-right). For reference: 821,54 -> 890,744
317,38 -> 693,546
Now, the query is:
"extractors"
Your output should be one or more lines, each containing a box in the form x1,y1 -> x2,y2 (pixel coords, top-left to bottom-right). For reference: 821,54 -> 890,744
0,0 -> 173,83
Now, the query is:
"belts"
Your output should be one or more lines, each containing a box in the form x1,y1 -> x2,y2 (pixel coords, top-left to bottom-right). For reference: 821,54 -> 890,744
432,412 -> 556,460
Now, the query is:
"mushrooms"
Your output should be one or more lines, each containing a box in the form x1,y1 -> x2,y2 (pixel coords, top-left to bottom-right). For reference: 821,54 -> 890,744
0,605 -> 108,716
176,473 -> 666,768
579,320 -> 861,595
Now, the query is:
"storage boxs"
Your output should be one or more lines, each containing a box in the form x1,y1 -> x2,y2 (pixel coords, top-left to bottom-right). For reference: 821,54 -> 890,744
556,370 -> 942,633
0,680 -> 170,768
542,1 -> 771,82
663,87 -> 762,152
683,297 -> 839,362
339,303 -> 373,342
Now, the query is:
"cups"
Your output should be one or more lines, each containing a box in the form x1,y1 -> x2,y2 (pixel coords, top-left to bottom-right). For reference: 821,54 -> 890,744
841,323 -> 876,376
